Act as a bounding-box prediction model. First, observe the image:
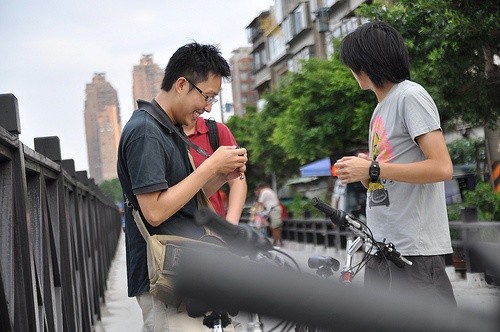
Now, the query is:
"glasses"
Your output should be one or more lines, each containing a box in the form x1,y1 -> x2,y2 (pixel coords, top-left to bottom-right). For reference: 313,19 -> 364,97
178,75 -> 218,106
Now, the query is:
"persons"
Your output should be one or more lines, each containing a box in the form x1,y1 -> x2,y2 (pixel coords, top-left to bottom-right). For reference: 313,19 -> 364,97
249,181 -> 284,250
180,115 -> 260,332
334,20 -> 458,312
115,41 -> 249,332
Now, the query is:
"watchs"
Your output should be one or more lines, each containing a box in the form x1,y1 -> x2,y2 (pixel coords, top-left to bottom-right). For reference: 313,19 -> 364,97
368,161 -> 380,183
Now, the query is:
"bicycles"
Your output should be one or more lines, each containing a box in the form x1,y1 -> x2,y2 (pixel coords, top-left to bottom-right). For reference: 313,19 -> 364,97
174,196 -> 500,332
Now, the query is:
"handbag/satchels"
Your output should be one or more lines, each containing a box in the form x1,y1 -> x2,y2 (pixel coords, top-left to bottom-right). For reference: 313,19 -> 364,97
146,233 -> 229,307
